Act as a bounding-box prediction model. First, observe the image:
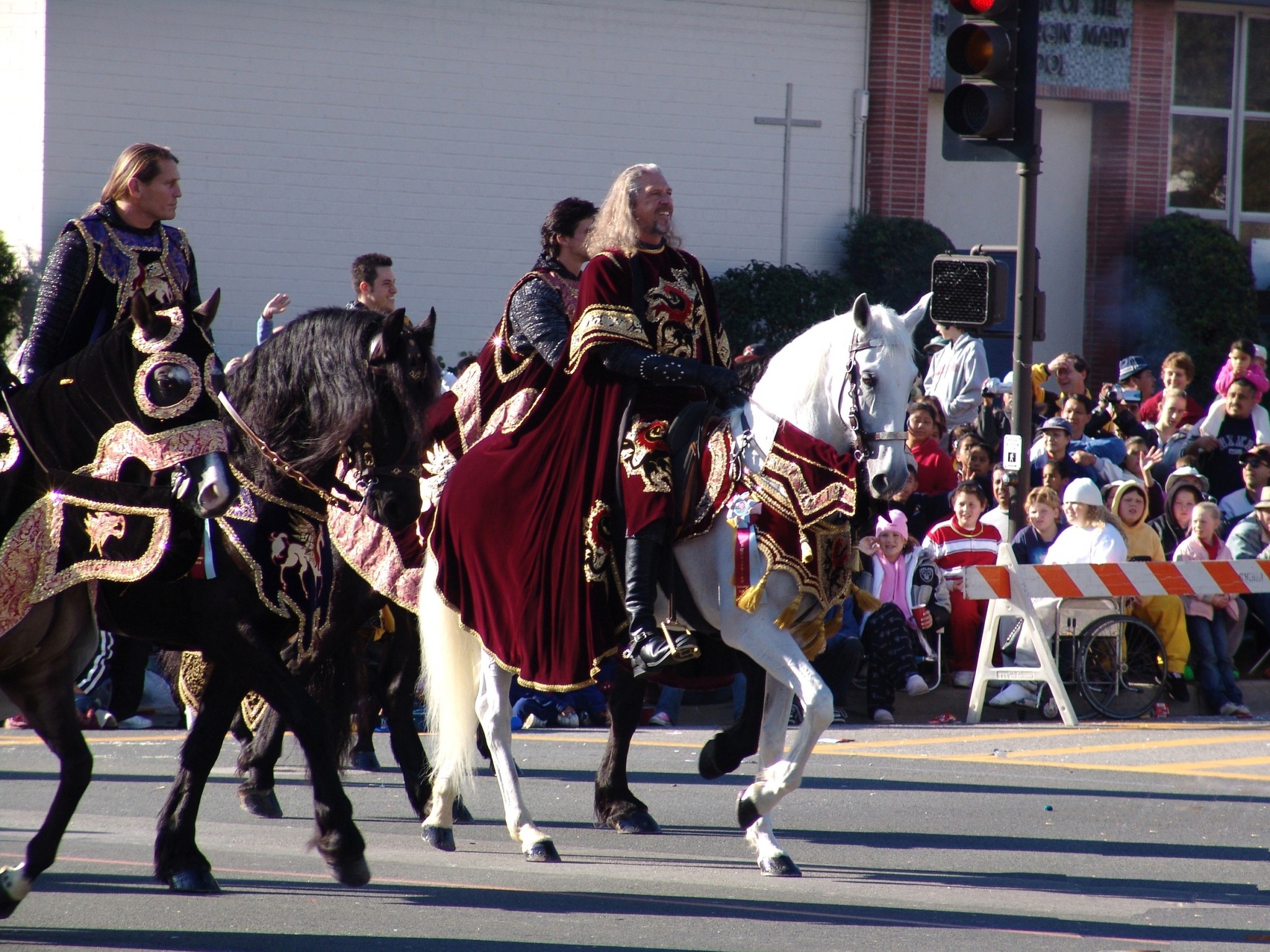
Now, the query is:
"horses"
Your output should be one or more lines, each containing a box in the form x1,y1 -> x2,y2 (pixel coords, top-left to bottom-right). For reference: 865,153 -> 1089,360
416,292 -> 924,877
0,285 -> 474,919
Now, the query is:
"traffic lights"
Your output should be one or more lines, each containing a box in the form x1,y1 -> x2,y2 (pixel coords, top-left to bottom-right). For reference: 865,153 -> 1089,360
930,254 -> 992,329
943,0 -> 1037,149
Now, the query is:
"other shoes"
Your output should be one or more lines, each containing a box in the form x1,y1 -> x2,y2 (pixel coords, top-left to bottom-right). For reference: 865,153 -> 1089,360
558,712 -> 581,727
1220,702 -> 1239,716
953,670 -> 975,688
987,683 -> 1037,706
521,713 -> 543,728
1154,666 -> 1191,703
873,709 -> 895,724
787,703 -> 802,725
651,711 -> 672,726
906,676 -> 930,697
120,715 -> 154,729
833,708 -> 848,724
1236,704 -> 1255,718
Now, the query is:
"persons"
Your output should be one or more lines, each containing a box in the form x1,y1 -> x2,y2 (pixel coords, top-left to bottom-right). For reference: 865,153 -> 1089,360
14,291 -> 774,731
23,141 -> 227,732
504,162 -> 756,681
739,299 -> 1270,726
349,253 -> 408,319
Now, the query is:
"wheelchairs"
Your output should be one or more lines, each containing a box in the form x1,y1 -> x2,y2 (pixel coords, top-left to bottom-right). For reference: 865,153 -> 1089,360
996,597 -> 1172,720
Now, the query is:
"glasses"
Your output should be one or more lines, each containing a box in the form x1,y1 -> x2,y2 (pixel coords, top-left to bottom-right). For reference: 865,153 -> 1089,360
1238,459 -> 1270,469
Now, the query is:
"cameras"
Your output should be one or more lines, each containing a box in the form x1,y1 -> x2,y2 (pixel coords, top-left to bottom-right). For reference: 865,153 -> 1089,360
1106,388 -> 1140,403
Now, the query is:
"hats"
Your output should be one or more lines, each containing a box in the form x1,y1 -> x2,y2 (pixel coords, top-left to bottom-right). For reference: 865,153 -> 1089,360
1252,486 -> 1270,508
1238,444 -> 1270,464
735,343 -> 780,364
1118,356 -> 1150,380
1099,480 -> 1124,495
874,509 -> 909,542
1036,417 -> 1073,435
1164,466 -> 1209,495
1253,343 -> 1267,361
1063,477 -> 1103,507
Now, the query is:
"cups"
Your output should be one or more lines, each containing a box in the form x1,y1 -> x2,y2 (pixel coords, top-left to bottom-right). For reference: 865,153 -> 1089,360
911,604 -> 929,628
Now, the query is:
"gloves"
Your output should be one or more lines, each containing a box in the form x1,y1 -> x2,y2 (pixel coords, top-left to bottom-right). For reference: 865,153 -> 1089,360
641,351 -> 736,403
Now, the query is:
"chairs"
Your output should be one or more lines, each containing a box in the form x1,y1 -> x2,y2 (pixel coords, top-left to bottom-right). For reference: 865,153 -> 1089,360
851,618 -> 945,694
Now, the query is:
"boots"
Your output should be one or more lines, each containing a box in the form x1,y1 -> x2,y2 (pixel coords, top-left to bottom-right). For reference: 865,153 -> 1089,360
623,539 -> 692,680
